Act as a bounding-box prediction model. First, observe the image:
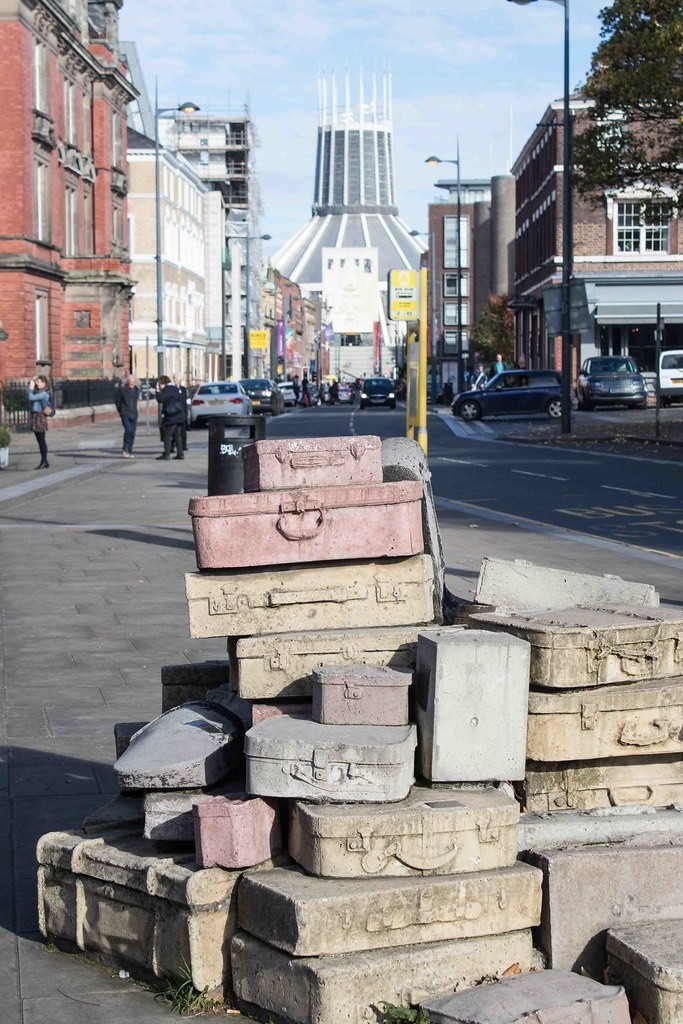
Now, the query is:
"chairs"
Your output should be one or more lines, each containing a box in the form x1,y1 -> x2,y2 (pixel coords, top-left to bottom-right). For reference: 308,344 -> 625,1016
202,389 -> 211,394
224,388 -> 236,392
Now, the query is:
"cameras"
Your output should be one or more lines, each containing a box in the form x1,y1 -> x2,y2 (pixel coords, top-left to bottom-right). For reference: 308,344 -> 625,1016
34,378 -> 37,382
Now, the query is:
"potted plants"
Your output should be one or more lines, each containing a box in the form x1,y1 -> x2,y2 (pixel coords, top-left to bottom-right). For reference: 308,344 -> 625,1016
0,428 -> 11,469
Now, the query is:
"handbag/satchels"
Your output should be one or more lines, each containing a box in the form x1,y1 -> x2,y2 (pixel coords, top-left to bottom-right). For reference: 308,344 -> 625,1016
164,401 -> 182,415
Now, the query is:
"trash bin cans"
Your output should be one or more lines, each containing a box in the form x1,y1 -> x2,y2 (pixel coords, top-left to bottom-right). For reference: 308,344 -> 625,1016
208,414 -> 265,495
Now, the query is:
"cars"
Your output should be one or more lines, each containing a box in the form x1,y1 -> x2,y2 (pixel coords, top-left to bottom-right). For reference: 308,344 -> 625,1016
238,378 -> 286,416
450,369 -> 579,422
190,381 -> 256,428
576,356 -> 647,411
359,378 -> 397,409
277,380 -> 356,405
638,371 -> 660,408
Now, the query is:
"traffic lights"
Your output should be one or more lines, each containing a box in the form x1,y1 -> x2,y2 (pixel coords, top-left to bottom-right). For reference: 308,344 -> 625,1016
302,366 -> 309,381
311,370 -> 317,382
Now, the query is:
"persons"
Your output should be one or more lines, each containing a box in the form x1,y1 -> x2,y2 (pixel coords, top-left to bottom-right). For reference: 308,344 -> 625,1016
328,382 -> 340,404
354,378 -> 362,405
300,376 -> 312,407
114,374 -> 139,458
293,375 -> 300,406
155,375 -> 189,459
27,374 -> 50,470
470,364 -> 487,388
493,353 -> 504,385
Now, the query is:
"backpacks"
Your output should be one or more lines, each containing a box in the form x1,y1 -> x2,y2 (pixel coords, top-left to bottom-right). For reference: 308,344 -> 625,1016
41,393 -> 55,417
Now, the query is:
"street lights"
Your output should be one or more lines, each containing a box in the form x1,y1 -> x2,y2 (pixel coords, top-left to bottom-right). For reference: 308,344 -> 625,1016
245,225 -> 272,376
408,229 -> 440,404
156,78 -> 201,373
507,0 -> 575,434
426,137 -> 468,394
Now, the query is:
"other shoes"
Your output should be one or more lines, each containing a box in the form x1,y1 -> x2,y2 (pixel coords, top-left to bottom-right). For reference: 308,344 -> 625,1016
170,448 -> 175,453
156,452 -> 170,460
130,454 -> 135,458
173,452 -> 184,459
183,447 -> 188,450
35,462 -> 49,469
123,448 -> 129,457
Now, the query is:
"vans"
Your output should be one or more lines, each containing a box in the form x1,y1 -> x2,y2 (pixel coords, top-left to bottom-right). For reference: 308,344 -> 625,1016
659,350 -> 683,407
